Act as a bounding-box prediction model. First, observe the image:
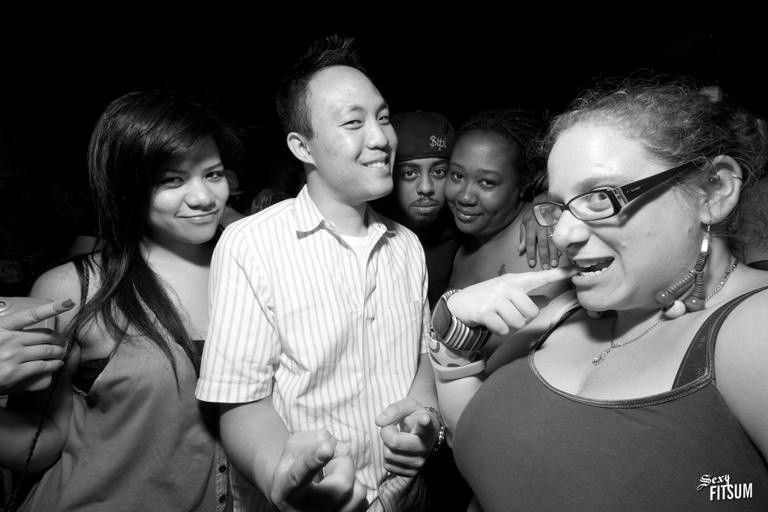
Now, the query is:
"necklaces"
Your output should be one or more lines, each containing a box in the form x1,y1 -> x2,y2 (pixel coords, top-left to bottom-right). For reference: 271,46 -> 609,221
592,256 -> 742,367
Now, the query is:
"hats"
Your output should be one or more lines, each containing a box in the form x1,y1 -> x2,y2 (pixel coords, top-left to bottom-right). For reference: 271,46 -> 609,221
389,108 -> 455,164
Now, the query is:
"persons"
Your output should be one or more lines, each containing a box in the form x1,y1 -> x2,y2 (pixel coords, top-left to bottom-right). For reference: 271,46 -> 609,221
190,29 -> 442,512
0,89 -> 279,512
33,126 -> 290,262
429,79 -> 768,511
251,110 -> 454,316
444,111 -> 570,289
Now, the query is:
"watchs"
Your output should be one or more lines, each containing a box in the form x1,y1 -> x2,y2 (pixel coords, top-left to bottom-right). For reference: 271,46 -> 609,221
424,406 -> 447,454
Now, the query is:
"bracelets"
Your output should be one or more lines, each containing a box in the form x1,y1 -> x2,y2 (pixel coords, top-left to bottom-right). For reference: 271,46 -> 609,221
427,288 -> 493,382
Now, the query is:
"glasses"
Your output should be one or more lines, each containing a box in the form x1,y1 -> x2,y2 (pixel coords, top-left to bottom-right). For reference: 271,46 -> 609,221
535,152 -> 710,228
